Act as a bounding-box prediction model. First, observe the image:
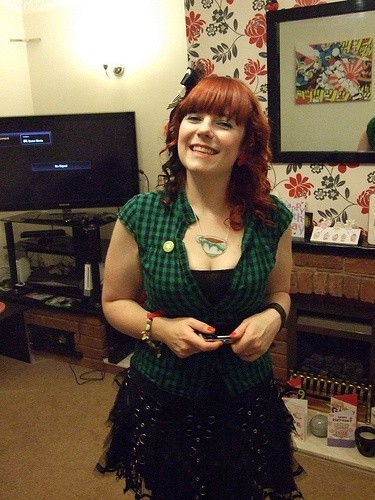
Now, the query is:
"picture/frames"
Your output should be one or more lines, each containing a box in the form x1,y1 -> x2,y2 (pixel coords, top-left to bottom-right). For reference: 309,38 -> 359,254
264,0 -> 375,167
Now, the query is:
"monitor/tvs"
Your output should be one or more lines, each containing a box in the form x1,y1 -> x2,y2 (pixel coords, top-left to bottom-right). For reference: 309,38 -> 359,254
0,111 -> 140,224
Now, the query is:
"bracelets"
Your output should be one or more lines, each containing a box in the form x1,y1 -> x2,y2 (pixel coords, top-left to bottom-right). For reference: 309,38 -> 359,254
142,318 -> 156,348
263,303 -> 287,330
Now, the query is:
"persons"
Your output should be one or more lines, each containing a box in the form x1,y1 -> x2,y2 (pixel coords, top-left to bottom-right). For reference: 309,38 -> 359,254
95,76 -> 307,500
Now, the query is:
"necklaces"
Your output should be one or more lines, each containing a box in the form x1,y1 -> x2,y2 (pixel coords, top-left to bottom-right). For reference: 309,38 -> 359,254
190,203 -> 231,258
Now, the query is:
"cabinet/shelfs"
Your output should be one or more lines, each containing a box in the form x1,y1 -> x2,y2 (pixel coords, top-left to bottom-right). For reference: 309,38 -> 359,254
0,209 -> 129,374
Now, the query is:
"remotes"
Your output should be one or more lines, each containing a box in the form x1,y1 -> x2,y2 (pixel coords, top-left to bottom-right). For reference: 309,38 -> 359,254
21,230 -> 65,238
